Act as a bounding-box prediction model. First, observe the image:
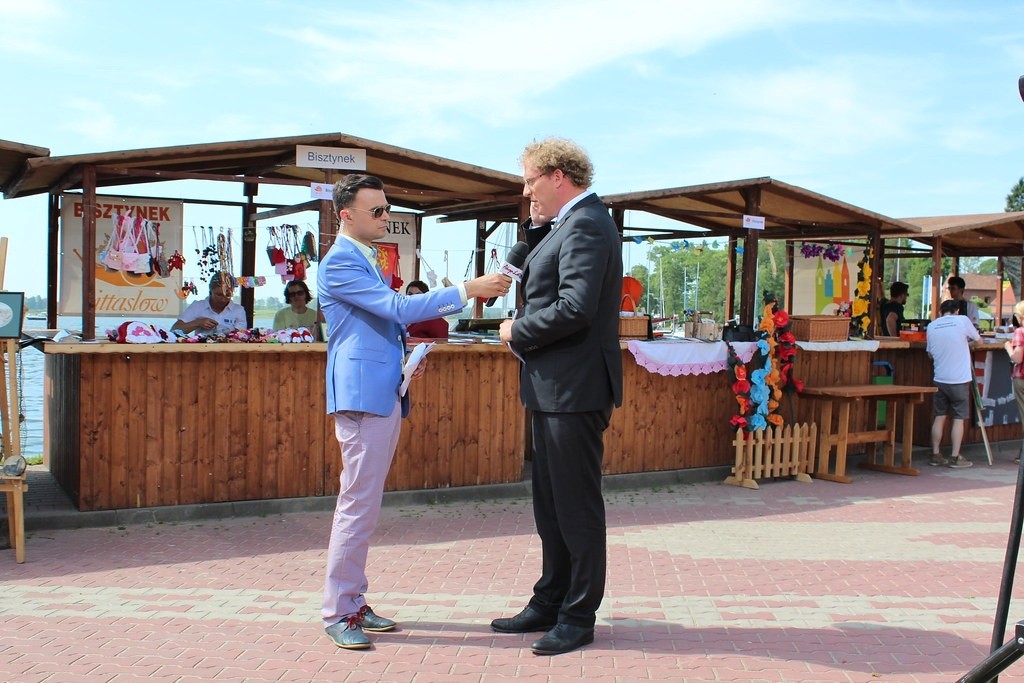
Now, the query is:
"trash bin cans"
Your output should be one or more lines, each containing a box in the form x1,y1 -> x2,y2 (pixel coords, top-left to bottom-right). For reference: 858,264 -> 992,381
872,360 -> 894,425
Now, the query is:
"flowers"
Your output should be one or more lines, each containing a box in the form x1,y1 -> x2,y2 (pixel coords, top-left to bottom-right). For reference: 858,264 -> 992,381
722,297 -> 805,437
797,240 -> 845,263
836,232 -> 874,340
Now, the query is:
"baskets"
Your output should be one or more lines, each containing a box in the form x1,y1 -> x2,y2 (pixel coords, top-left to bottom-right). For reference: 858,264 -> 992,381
618,293 -> 648,341
788,313 -> 851,342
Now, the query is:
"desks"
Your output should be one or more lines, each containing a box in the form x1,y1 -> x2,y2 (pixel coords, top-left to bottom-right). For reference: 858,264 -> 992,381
796,384 -> 938,485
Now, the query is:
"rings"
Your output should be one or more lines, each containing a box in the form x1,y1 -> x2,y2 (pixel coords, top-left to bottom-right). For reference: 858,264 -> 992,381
502,288 -> 506,292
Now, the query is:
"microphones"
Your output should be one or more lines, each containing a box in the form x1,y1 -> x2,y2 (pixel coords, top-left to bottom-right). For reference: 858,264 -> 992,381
485,240 -> 529,308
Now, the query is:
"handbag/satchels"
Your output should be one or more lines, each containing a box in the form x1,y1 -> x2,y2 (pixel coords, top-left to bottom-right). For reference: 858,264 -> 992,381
99,214 -> 170,279
267,224 -> 310,283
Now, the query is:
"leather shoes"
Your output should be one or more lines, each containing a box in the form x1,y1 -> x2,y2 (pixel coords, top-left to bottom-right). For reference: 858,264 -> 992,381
531,623 -> 595,654
490,606 -> 557,633
324,615 -> 371,647
354,604 -> 397,630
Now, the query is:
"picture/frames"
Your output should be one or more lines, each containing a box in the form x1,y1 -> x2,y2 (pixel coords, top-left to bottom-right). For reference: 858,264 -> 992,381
0,292 -> 24,338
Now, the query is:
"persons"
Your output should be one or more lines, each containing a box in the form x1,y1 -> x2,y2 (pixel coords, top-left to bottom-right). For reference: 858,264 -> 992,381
882,281 -> 910,337
169,272 -> 247,336
317,174 -> 512,652
406,280 -> 449,339
1004,301 -> 1024,464
491,134 -> 624,656
948,276 -> 979,328
926,300 -> 983,468
273,280 -> 317,331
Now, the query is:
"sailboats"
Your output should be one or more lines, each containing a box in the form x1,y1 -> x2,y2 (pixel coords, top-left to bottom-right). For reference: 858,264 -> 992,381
647,247 -> 715,338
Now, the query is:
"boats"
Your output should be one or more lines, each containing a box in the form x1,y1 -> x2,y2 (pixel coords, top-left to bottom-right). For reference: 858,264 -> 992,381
26,316 -> 46,320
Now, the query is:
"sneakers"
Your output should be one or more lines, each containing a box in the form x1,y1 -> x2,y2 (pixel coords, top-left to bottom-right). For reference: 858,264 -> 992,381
929,453 -> 949,465
949,452 -> 973,468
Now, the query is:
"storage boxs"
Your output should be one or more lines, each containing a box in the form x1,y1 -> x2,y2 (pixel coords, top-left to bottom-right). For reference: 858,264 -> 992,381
993,326 -> 1015,339
899,330 -> 926,342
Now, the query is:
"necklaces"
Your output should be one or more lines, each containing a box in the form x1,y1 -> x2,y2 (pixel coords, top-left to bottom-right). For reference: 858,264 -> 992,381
192,226 -> 234,298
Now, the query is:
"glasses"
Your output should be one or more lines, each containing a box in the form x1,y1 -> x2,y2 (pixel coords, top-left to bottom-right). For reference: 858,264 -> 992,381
948,286 -> 961,291
286,289 -> 306,299
523,168 -> 566,188
347,203 -> 391,218
903,293 -> 909,296
